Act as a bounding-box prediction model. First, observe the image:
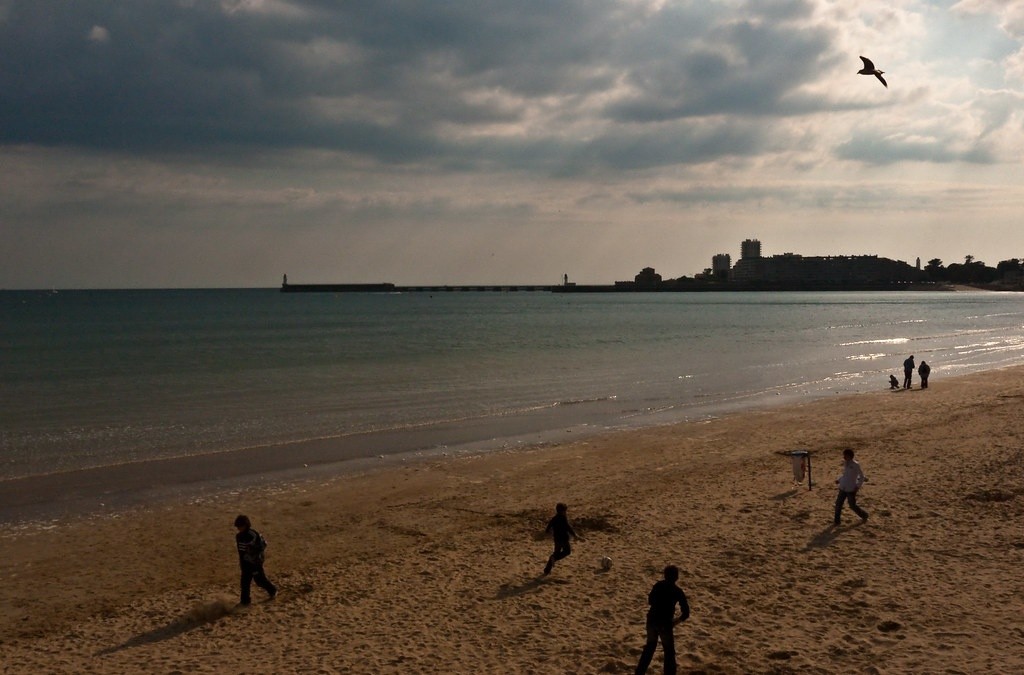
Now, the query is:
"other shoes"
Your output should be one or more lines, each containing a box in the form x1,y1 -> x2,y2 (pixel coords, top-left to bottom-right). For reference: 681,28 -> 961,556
272,590 -> 278,598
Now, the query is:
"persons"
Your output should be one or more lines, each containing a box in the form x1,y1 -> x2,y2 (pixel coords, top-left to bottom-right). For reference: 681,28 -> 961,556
635,565 -> 690,675
543,501 -> 586,575
233,514 -> 278,605
832,448 -> 868,526
888,374 -> 899,389
903,354 -> 916,389
918,360 -> 930,389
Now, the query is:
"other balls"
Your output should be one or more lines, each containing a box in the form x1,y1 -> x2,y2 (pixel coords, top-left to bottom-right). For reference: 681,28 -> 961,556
600,556 -> 614,570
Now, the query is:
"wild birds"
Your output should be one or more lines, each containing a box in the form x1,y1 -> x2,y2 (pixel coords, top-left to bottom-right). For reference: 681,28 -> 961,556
857,56 -> 888,90
